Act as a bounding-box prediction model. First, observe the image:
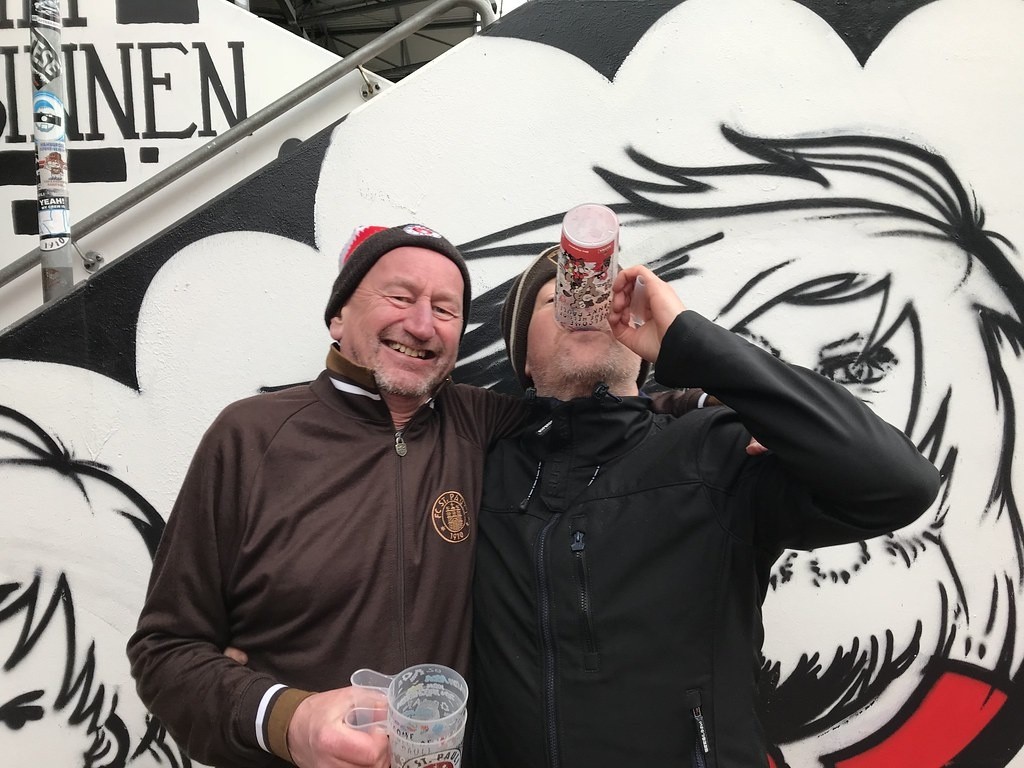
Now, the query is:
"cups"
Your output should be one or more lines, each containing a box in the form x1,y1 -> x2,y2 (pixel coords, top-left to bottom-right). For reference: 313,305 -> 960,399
554,203 -> 645,333
346,663 -> 468,768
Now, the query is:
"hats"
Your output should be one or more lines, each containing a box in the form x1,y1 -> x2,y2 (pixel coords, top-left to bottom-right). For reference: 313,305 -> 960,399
325,225 -> 471,348
500,246 -> 650,392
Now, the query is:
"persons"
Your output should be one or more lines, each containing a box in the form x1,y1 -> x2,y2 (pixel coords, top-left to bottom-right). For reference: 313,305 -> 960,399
222,239 -> 942,767
123,221 -> 769,768
